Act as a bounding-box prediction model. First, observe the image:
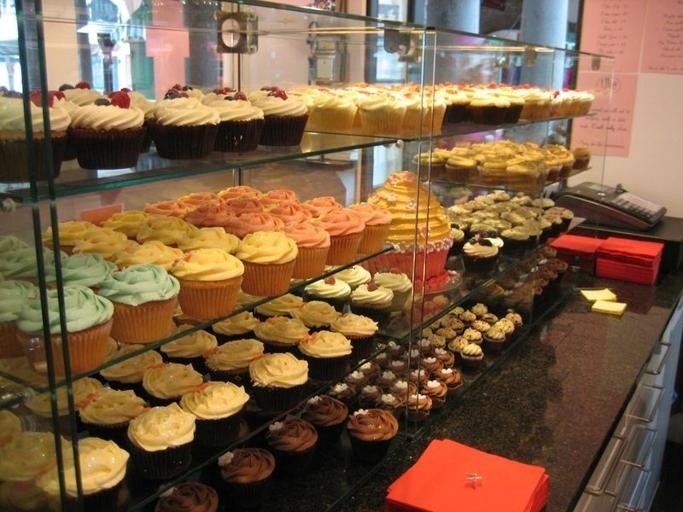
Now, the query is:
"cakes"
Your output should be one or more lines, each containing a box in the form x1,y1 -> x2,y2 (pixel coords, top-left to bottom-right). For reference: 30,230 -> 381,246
201,338 -> 264,389
302,278 -> 351,313
0,91 -> 71,183
296,330 -> 354,380
204,93 -> 264,150
0,431 -> 67,508
420,378 -> 448,409
348,283 -> 393,326
327,382 -> 357,406
141,363 -> 203,408
35,436 -> 131,512
0,280 -> 49,359
94,263 -> 181,344
72,228 -> 137,263
447,190 -> 573,275
234,232 -> 299,296
182,203 -> 235,230
49,90 -> 80,116
68,92 -> 149,170
106,87 -> 152,112
388,377 -> 418,400
345,408 -> 398,466
278,223 -> 330,279
59,82 -> 103,107
225,211 -> 283,240
375,392 -> 406,421
178,380 -> 251,439
402,348 -> 421,369
252,316 -> 309,361
384,357 -> 408,379
170,247 -> 243,319
154,483 -> 225,512
355,385 -> 383,408
168,84 -> 205,107
370,351 -> 391,371
159,324 -> 217,377
249,85 -> 279,104
412,139 -> 591,188
216,446 -> 275,512
347,202 -> 392,254
249,293 -> 305,322
175,225 -> 243,259
297,394 -> 349,450
325,263 -> 372,307
210,303 -> 260,346
372,271 -> 410,311
385,341 -> 403,361
22,376 -> 103,433
357,358 -> 381,382
250,90 -> 309,148
343,370 -> 368,391
310,207 -> 366,266
434,367 -> 466,396
134,215 -> 199,250
126,402 -> 197,481
0,408 -> 22,448
261,414 -> 319,478
112,239 -> 184,278
144,184 -> 298,214
14,284 -> 114,376
417,295 -> 522,369
287,300 -> 342,336
143,87 -> 221,161
248,352 -> 308,410
44,252 -> 120,295
0,233 -> 27,253
403,394 -> 433,425
373,368 -> 399,395
0,247 -> 69,286
98,345 -> 163,394
348,170 -> 454,290
267,203 -> 313,228
481,244 -> 568,310
99,210 -> 154,244
328,312 -> 379,359
202,86 -> 236,106
406,366 -> 430,393
77,385 -> 146,448
41,221 -> 99,257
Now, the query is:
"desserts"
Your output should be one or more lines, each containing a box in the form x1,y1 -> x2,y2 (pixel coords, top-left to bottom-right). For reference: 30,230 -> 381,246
292,82 -> 594,137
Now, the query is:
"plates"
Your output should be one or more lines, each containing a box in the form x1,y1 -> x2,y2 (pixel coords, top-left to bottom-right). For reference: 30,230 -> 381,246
413,272 -> 461,294
